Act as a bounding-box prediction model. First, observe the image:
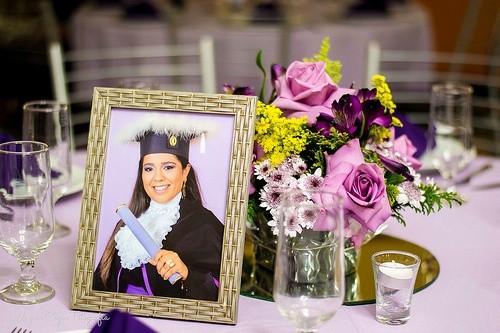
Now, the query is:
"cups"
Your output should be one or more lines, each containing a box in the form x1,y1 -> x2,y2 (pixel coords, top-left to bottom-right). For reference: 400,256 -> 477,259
371,250 -> 421,325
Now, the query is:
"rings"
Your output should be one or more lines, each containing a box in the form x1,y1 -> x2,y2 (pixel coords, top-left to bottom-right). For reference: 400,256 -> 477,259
165,259 -> 174,267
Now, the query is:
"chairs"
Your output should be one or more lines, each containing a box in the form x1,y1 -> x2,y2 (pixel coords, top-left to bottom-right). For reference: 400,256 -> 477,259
48,34 -> 218,151
363,42 -> 500,156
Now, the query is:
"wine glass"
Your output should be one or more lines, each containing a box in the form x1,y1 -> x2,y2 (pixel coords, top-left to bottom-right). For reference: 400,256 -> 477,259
428,84 -> 477,205
273,190 -> 345,333
0,140 -> 56,305
22,101 -> 73,240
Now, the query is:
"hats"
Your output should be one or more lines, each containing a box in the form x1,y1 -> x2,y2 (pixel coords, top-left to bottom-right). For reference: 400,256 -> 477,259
114,114 -> 217,161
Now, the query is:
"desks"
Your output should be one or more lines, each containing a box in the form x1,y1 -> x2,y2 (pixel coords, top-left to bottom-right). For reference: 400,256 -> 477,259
75,7 -> 436,103
0,150 -> 500,333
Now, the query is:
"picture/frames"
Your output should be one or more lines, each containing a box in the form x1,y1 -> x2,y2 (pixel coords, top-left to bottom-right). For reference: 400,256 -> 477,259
69,87 -> 259,325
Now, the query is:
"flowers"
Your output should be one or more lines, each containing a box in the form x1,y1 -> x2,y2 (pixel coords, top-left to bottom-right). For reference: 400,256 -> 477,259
213,34 -> 468,256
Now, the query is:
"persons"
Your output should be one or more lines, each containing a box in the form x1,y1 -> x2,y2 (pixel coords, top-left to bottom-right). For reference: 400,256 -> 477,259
92,116 -> 221,303
